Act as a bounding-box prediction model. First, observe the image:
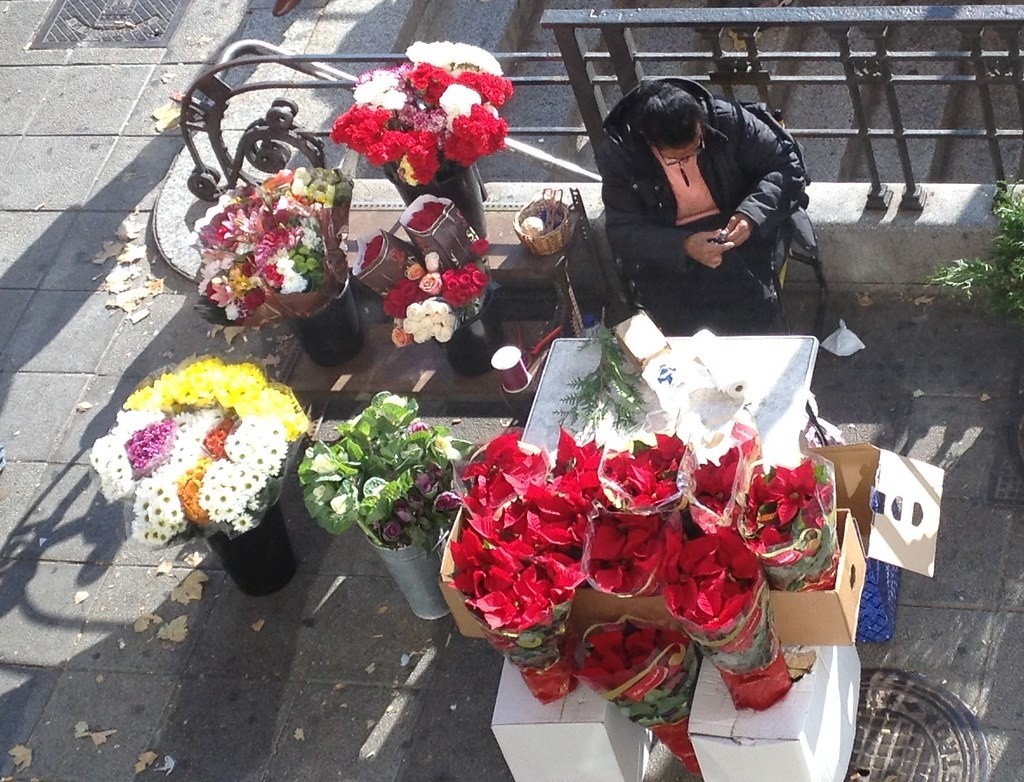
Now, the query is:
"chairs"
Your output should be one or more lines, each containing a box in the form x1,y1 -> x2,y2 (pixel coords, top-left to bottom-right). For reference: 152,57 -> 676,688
774,106 -> 830,338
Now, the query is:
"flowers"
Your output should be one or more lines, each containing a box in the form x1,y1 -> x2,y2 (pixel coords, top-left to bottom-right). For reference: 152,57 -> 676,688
92,41 -> 840,776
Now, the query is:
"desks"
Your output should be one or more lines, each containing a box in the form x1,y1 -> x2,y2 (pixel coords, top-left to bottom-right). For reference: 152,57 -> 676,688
521,332 -> 829,468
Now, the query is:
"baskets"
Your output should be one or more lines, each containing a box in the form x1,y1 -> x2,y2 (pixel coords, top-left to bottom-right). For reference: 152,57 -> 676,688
513,199 -> 571,256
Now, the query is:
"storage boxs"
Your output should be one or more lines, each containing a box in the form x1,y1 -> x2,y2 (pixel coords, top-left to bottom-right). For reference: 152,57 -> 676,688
803,441 -> 945,645
491,657 -> 654,782
437,501 -> 867,648
684,644 -> 862,782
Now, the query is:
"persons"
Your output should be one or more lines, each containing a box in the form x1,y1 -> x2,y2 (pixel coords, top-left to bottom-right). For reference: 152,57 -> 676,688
603,77 -> 806,331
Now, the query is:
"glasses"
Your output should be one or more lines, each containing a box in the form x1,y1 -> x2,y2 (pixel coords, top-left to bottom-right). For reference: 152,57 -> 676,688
655,134 -> 705,166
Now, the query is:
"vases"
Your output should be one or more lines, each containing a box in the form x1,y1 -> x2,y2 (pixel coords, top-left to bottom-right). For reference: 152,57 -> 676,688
431,288 -> 503,377
287,267 -> 363,369
382,153 -> 489,239
205,503 -> 295,597
364,528 -> 451,620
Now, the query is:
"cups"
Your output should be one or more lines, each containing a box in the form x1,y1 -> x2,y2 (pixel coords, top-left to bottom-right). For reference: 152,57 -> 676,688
491,345 -> 530,393
